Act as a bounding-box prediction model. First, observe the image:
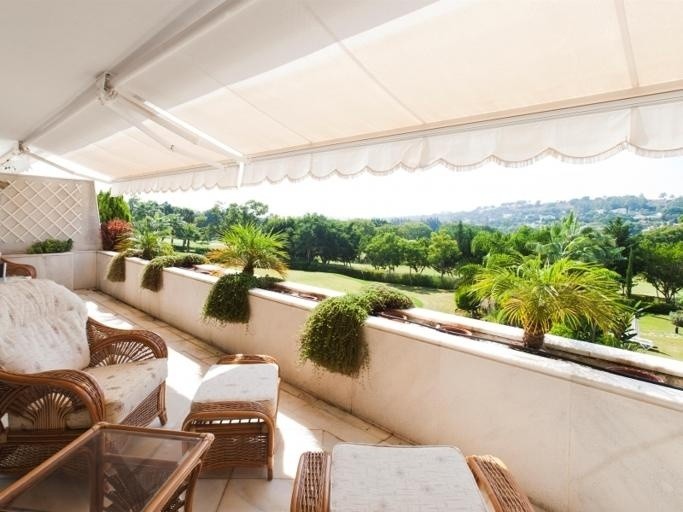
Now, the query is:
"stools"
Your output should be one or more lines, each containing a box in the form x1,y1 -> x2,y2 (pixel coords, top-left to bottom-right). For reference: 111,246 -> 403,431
289,442 -> 532,512
178,352 -> 282,482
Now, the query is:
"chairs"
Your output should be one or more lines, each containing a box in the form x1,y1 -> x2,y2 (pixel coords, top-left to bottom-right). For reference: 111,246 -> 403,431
0,276 -> 170,494
0,257 -> 37,279
